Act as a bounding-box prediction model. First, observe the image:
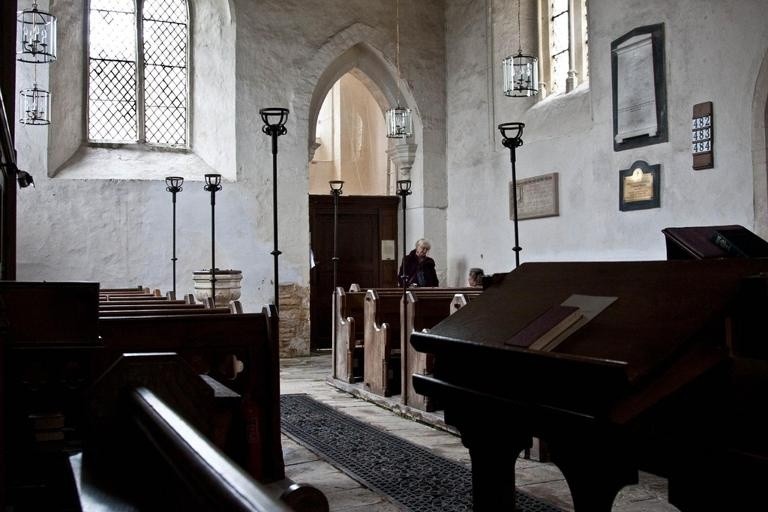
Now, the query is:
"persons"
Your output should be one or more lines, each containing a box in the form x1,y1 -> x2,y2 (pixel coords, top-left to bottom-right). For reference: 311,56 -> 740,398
468,267 -> 485,288
397,238 -> 441,289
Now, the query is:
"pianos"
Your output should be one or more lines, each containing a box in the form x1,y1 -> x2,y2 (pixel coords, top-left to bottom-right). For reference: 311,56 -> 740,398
410,225 -> 766,512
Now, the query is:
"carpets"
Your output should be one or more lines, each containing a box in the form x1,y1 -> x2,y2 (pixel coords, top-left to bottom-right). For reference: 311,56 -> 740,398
279,393 -> 567,512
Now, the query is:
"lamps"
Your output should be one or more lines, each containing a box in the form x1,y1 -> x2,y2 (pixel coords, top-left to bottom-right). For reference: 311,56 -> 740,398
14,0 -> 56,125
385,0 -> 414,139
501,0 -> 539,96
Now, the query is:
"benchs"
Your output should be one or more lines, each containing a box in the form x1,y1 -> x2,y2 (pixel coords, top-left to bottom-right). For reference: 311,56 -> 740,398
331,283 -> 482,438
65,286 -> 329,512
